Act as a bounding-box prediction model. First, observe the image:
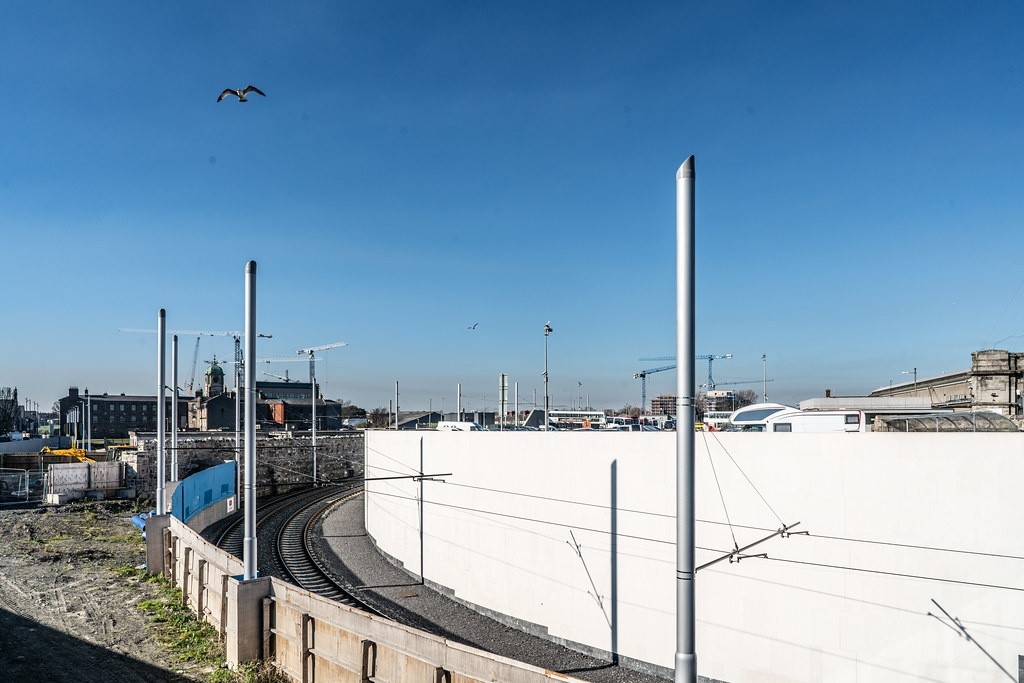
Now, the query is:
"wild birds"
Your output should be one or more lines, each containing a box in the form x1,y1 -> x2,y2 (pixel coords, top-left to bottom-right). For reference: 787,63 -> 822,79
216,83 -> 266,104
467,322 -> 479,330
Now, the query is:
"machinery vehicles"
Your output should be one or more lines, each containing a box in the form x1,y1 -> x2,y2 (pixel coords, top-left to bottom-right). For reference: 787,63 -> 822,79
38,446 -> 139,464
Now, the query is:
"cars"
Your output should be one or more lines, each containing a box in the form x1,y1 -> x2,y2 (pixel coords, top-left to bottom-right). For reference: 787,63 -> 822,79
415,396 -> 868,434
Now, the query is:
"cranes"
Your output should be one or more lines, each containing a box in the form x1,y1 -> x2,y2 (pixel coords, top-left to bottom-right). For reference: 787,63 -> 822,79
699,379 -> 773,391
261,370 -> 297,382
296,341 -> 348,385
118,326 -> 272,389
639,354 -> 734,392
206,353 -> 323,399
633,365 -> 676,410
